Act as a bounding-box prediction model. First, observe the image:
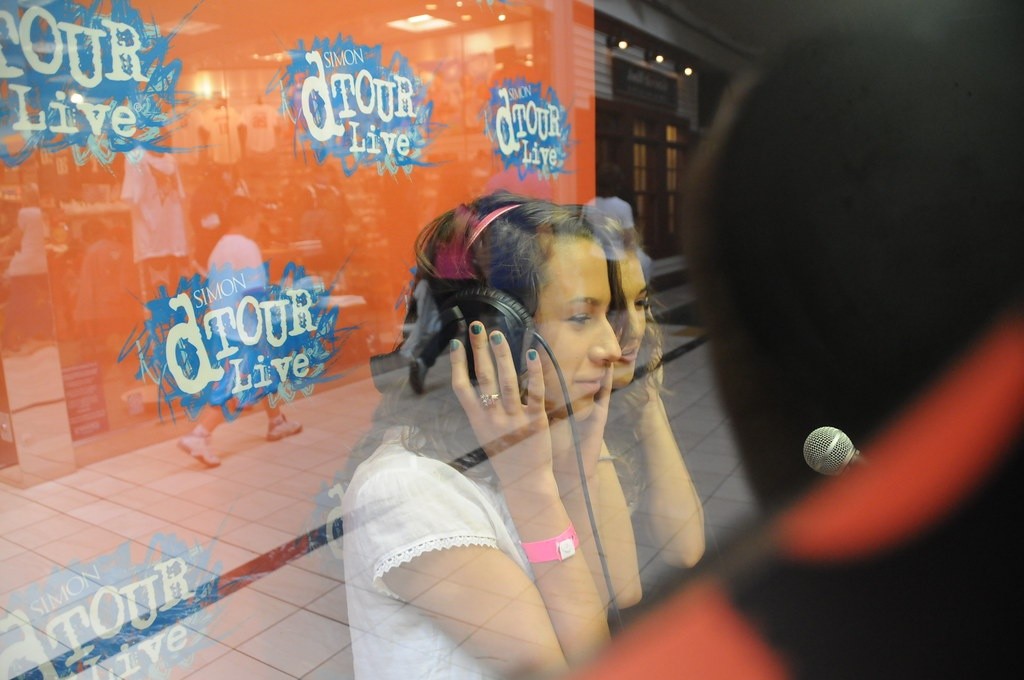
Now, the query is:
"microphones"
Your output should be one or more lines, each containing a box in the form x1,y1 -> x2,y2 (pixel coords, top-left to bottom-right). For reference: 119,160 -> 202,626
802,426 -> 862,474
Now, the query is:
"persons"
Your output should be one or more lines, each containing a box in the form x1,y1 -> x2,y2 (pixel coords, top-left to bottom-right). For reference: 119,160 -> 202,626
175,197 -> 303,468
332,190 -> 707,680
0,184 -> 56,348
117,122 -> 190,331
563,23 -> 1024,679
575,158 -> 637,265
76,218 -> 128,318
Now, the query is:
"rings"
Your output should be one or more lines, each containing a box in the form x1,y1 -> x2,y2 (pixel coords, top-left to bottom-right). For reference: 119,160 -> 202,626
479,393 -> 501,407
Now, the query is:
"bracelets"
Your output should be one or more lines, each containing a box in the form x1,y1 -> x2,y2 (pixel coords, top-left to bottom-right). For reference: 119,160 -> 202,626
519,521 -> 579,564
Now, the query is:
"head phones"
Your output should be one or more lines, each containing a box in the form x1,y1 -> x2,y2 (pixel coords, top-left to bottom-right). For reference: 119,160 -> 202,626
411,201 -> 535,376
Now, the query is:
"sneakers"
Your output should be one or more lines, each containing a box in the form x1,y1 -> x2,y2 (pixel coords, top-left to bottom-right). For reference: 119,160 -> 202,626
266,413 -> 303,441
178,433 -> 220,467
408,359 -> 430,395
